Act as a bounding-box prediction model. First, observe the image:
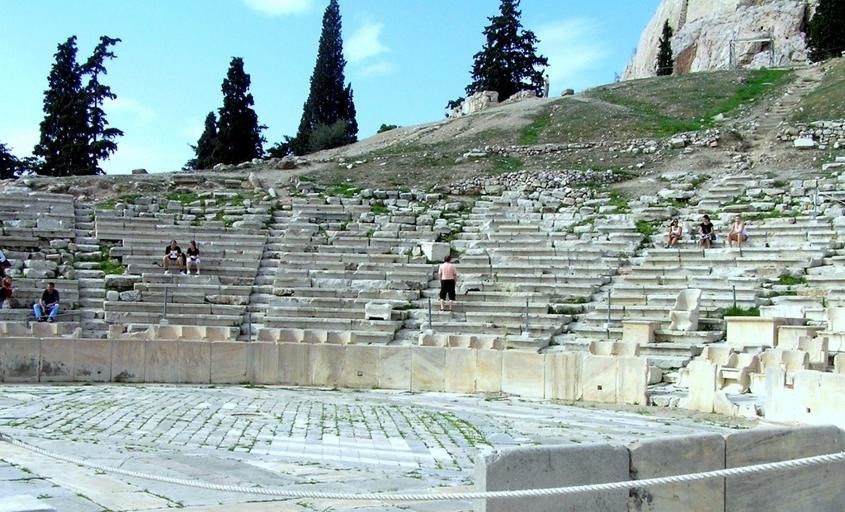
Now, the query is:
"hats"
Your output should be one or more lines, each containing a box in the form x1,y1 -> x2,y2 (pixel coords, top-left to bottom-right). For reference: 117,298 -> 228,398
1,276 -> 12,289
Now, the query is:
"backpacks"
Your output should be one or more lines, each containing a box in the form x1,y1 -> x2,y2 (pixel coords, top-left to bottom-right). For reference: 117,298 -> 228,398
182,253 -> 186,265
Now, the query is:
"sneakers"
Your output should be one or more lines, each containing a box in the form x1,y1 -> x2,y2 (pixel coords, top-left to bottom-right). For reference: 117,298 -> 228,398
164,269 -> 169,275
195,272 -> 200,274
180,270 -> 191,275
38,317 -> 53,322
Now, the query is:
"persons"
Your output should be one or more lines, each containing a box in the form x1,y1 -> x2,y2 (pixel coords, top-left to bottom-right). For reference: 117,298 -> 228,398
543,74 -> 549,98
0,248 -> 13,309
727,215 -> 748,247
163,240 -> 186,275
698,215 -> 713,249
438,256 -> 456,311
186,241 -> 201,275
664,218 -> 682,248
33,282 -> 59,322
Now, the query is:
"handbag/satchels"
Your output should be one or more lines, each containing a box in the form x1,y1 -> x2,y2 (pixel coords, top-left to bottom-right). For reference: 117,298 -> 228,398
0,260 -> 11,269
711,233 -> 716,240
